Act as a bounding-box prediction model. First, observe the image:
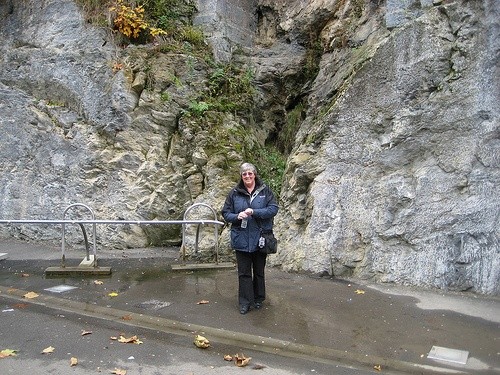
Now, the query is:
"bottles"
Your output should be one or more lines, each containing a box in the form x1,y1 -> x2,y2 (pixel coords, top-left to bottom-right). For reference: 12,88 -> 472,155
241,214 -> 248,228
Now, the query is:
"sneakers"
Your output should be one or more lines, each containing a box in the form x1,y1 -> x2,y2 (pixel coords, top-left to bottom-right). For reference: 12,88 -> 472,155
253,302 -> 260,309
240,304 -> 250,314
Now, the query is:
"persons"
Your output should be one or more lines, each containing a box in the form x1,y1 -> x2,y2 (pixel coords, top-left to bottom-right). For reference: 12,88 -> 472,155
220,163 -> 278,315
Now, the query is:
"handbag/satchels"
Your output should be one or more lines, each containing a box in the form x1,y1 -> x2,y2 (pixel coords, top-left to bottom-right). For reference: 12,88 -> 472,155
258,233 -> 277,254
